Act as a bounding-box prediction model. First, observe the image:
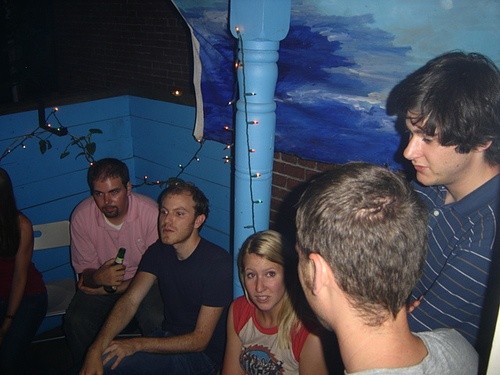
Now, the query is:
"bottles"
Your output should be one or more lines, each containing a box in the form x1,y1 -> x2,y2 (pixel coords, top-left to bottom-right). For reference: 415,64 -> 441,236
104,248 -> 126,292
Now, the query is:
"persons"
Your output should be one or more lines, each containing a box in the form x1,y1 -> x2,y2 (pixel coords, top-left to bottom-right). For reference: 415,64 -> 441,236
79,183 -> 233,375
385,50 -> 500,351
291,161 -> 479,375
0,168 -> 48,375
59,157 -> 161,374
223,230 -> 329,375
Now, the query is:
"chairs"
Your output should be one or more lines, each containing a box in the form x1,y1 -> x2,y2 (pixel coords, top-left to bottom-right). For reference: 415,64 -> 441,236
27,220 -> 73,345
